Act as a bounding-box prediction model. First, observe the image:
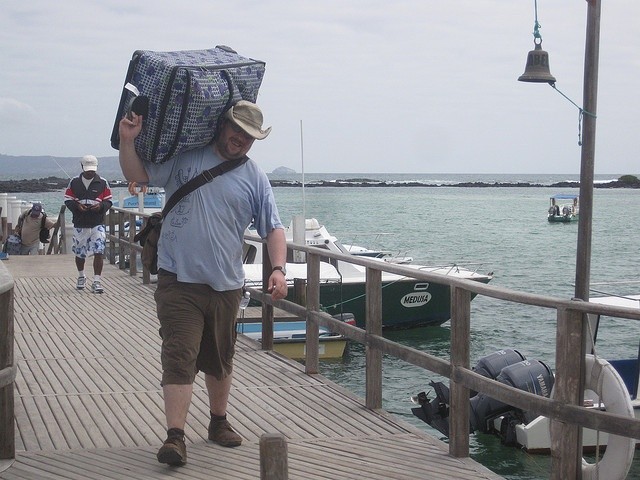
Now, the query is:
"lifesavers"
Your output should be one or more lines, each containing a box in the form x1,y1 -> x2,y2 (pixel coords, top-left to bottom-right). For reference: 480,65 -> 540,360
550,355 -> 635,480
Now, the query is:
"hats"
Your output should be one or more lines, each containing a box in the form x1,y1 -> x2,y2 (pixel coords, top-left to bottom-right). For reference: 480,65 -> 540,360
81,155 -> 98,172
33,204 -> 41,215
225,100 -> 272,140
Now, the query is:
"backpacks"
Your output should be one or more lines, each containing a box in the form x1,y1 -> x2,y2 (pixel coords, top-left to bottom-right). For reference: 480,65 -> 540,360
28,209 -> 51,243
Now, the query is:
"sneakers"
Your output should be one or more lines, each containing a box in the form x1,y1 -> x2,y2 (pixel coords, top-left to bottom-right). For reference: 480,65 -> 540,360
77,277 -> 86,290
91,281 -> 104,294
157,430 -> 187,467
208,417 -> 243,447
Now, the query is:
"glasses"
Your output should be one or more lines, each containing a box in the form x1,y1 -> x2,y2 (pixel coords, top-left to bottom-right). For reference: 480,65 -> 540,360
228,119 -> 252,139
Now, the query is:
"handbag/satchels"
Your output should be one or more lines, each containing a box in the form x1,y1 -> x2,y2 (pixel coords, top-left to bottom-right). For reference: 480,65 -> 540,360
134,155 -> 250,274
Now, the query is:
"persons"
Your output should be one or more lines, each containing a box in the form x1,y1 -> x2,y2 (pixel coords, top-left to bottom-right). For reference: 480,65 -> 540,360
116,100 -> 289,466
64,155 -> 113,293
14,202 -> 58,255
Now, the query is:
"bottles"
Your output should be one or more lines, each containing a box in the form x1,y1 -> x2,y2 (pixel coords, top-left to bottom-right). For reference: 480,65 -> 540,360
241,292 -> 250,309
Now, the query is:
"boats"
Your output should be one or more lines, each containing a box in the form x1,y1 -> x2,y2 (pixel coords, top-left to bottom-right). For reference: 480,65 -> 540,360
238,310 -> 359,363
410,291 -> 640,435
547,190 -> 584,225
234,118 -> 416,264
94,184 -> 167,233
213,256 -> 495,331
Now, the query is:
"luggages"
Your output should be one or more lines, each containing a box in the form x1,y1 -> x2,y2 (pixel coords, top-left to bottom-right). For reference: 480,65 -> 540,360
3,233 -> 22,255
110,45 -> 266,164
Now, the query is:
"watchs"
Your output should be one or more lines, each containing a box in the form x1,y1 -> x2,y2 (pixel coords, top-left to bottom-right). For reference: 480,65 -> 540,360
272,266 -> 287,276
